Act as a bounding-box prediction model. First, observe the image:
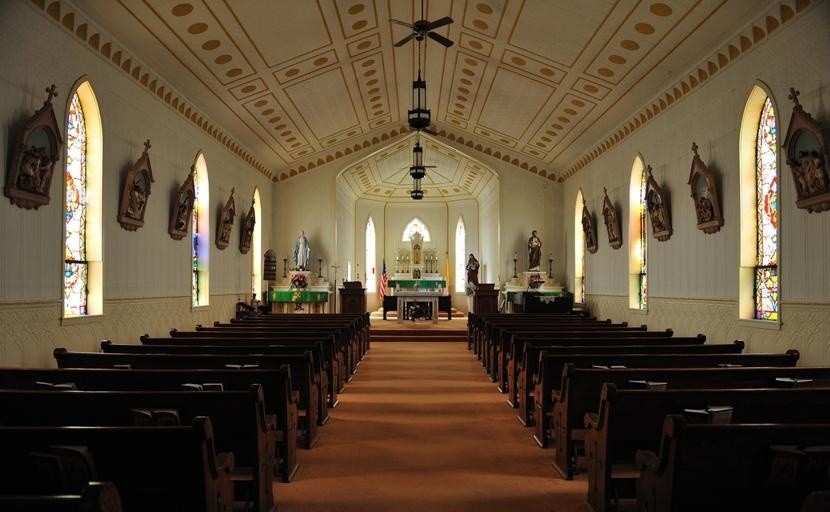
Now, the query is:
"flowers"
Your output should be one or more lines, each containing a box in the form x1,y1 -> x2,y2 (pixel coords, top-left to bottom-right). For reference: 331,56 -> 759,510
286,272 -> 308,290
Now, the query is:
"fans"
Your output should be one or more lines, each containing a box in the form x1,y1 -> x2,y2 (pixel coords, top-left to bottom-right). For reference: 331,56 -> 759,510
389,1 -> 458,48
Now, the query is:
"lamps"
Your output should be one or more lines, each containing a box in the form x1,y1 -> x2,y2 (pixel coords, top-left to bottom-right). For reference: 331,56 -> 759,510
407,37 -> 435,200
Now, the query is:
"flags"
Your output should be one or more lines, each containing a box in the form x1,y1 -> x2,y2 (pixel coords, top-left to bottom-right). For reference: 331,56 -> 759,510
379,258 -> 387,300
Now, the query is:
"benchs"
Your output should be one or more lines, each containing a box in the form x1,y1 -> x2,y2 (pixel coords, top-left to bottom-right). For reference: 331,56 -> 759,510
0,309 -> 374,511
465,308 -> 830,511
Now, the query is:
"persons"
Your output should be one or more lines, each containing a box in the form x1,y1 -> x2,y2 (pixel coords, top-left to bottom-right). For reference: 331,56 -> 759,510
16,144 -> 55,195
528,230 -> 541,271
466,254 -> 480,284
292,230 -> 312,272
784,147 -> 830,198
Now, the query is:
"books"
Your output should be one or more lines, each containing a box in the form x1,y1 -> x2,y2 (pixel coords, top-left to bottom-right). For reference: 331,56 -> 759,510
797,378 -> 813,386
685,408 -> 709,426
131,406 -> 154,426
35,380 -> 50,389
150,410 -> 181,426
591,364 -> 607,370
708,405 -> 733,424
776,376 -> 794,387
648,381 -> 668,391
205,382 -> 224,391
243,364 -> 262,369
610,365 -> 626,369
113,363 -> 132,368
182,383 -> 203,391
54,383 -> 78,390
224,363 -> 241,369
627,379 -> 647,390
